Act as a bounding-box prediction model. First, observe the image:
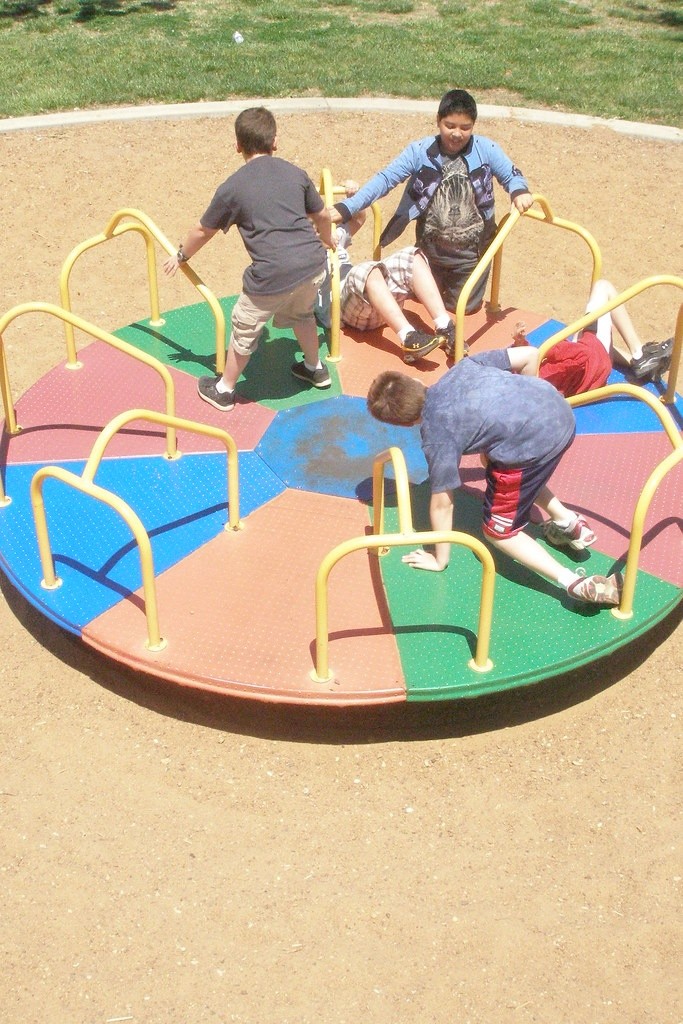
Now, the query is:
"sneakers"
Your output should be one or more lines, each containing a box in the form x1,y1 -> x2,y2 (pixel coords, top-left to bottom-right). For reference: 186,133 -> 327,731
401,331 -> 446,365
630,336 -> 676,382
543,513 -> 596,550
290,355 -> 330,387
567,568 -> 626,606
433,316 -> 470,357
198,374 -> 236,411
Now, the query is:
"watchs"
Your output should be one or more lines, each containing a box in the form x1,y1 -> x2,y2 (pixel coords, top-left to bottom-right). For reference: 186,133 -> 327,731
177,249 -> 189,262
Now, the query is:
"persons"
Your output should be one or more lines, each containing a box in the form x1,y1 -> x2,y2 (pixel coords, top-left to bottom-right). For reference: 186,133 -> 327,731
164,108 -> 338,410
513,280 -> 673,398
314,181 -> 470,363
367,346 -> 625,605
327,89 -> 533,315
